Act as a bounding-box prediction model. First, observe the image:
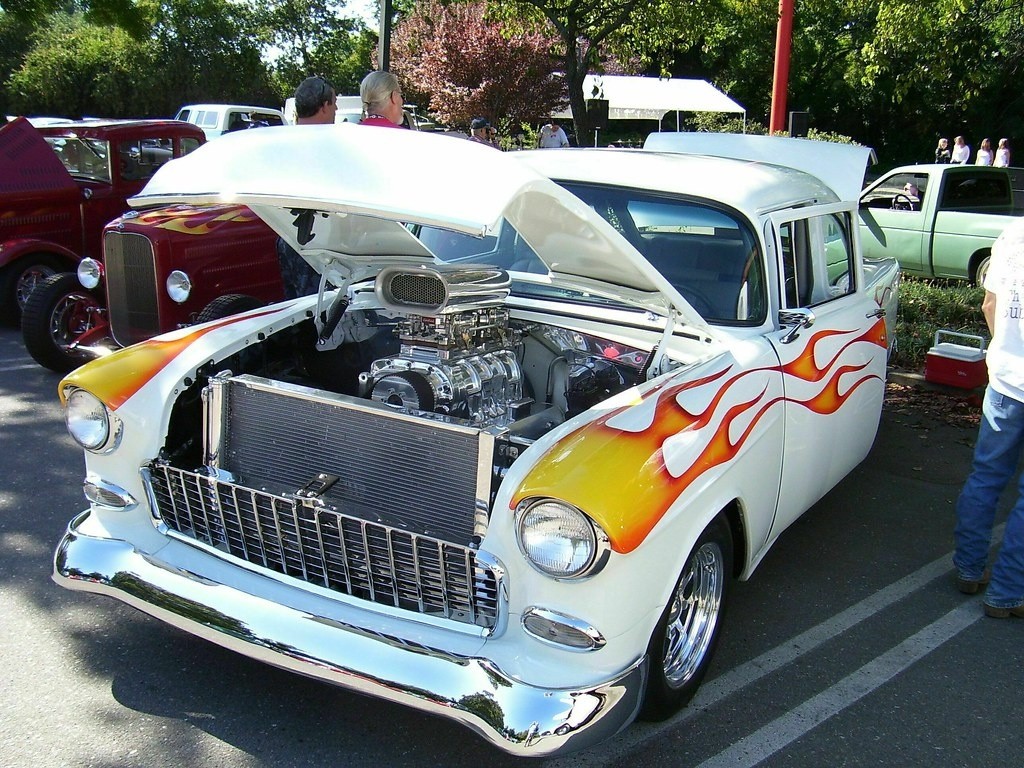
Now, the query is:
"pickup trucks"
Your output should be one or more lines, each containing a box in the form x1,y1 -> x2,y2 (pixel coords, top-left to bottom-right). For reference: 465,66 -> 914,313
856,163 -> 1024,293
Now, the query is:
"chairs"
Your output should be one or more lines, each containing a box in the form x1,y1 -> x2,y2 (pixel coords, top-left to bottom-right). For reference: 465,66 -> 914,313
650,235 -> 743,283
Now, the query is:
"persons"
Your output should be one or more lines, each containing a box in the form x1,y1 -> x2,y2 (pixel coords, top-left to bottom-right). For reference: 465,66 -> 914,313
357,71 -> 407,128
904,183 -> 925,201
993,138 -> 1010,167
953,216 -> 1024,618
950,136 -> 970,164
539,118 -> 569,149
294,76 -> 338,125
975,138 -> 994,166
468,118 -> 500,150
935,139 -> 952,164
248,111 -> 269,128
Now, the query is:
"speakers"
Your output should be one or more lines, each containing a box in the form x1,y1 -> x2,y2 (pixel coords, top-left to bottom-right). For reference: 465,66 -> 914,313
586,99 -> 608,129
789,111 -> 809,137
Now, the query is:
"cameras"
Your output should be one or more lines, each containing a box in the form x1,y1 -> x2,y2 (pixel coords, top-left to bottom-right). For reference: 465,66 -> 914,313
486,125 -> 491,134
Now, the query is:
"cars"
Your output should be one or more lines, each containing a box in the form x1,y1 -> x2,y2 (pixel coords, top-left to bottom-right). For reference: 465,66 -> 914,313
51,123 -> 903,760
1,81 -> 418,374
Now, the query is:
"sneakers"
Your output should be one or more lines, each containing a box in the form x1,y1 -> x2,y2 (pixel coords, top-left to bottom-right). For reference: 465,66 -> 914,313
985,604 -> 1024,618
957,567 -> 989,594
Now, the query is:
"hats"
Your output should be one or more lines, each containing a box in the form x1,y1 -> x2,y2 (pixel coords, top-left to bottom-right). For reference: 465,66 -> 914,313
471,118 -> 490,129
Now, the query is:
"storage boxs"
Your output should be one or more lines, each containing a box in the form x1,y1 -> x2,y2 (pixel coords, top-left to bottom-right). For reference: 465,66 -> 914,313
925,331 -> 988,390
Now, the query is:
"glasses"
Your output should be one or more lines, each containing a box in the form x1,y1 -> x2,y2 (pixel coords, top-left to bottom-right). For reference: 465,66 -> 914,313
397,92 -> 406,103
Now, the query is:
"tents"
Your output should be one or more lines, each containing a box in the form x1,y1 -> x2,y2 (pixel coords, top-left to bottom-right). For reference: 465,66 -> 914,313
546,71 -> 745,147
